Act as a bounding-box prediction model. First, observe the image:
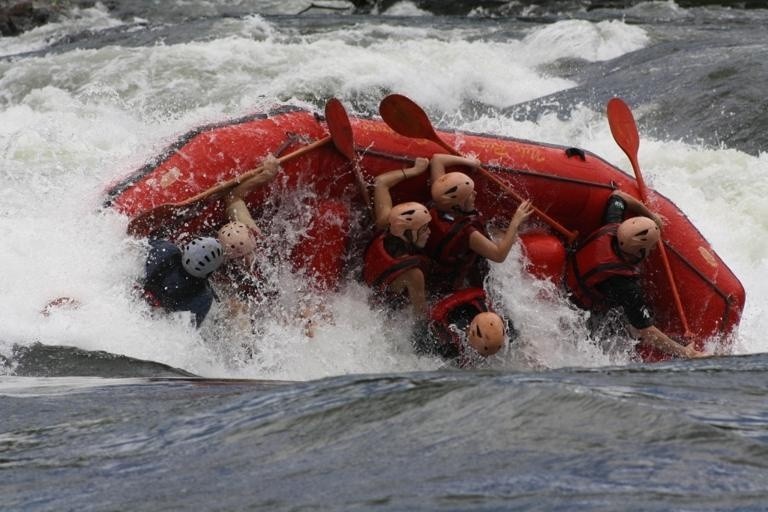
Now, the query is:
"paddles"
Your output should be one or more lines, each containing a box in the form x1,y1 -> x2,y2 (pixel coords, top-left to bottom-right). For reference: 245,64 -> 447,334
379,94 -> 571,238
606,97 -> 702,354
128,137 -> 329,239
325,98 -> 376,224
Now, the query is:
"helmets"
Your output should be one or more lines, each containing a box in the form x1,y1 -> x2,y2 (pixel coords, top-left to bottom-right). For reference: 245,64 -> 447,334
215,222 -> 257,259
617,215 -> 660,258
387,202 -> 432,242
181,237 -> 224,279
431,171 -> 475,212
468,311 -> 505,357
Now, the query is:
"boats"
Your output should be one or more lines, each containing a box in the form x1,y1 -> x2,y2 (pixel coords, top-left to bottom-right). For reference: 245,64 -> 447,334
89,103 -> 745,364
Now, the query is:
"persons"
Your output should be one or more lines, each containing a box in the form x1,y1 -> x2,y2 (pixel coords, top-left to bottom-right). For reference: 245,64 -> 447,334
346,154 -> 534,364
142,154 -> 281,319
564,191 -> 704,363
